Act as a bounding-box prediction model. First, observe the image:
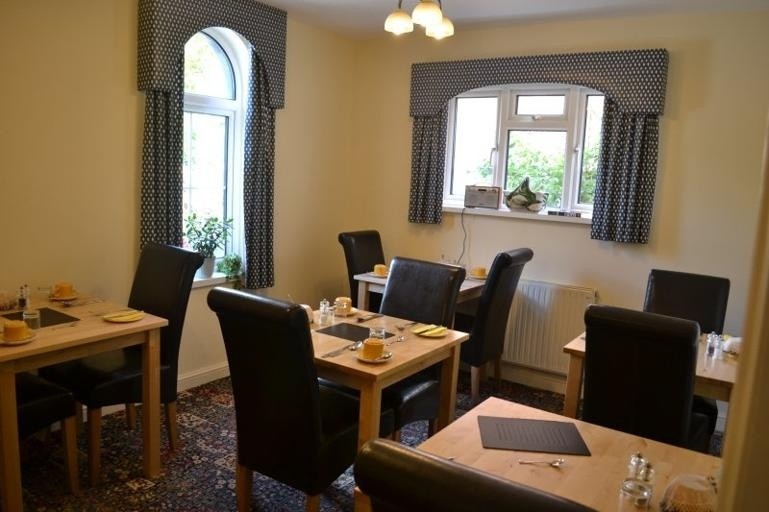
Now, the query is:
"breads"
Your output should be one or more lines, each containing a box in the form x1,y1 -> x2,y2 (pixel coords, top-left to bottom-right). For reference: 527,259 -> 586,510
1,319 -> 29,341
362,338 -> 384,359
335,297 -> 351,313
473,266 -> 486,276
55,282 -> 72,298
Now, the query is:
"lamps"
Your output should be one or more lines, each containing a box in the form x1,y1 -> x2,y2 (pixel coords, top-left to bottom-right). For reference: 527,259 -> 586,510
381,2 -> 459,43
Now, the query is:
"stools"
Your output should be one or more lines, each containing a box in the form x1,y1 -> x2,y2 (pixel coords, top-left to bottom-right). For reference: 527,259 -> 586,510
16,367 -> 80,494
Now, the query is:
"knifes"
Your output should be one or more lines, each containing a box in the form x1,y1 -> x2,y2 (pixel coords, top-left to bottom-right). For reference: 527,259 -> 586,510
415,325 -> 440,335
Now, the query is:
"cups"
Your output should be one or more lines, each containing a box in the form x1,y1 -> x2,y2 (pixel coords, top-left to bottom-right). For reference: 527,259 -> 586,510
471,266 -> 486,276
364,327 -> 386,360
0,284 -> 40,347
51,282 -> 73,297
617,477 -> 653,512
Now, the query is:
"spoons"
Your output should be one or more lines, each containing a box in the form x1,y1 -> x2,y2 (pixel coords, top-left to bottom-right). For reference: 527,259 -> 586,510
322,341 -> 362,358
519,458 -> 568,466
353,312 -> 384,322
61,298 -> 101,304
386,336 -> 408,346
396,322 -> 419,331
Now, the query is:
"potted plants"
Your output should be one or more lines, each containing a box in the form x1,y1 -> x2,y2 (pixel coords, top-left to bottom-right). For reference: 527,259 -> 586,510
183,209 -> 237,280
503,174 -> 548,214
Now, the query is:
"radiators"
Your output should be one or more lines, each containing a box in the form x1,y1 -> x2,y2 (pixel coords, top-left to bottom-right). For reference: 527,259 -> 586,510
502,277 -> 599,377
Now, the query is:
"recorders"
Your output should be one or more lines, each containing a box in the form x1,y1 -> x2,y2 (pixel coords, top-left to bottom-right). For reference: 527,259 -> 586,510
464,185 -> 503,209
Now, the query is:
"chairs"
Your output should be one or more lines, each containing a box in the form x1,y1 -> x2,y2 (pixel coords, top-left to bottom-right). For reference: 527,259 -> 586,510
642,267 -> 731,441
337,230 -> 391,312
316,254 -> 467,444
352,436 -> 598,512
34,238 -> 207,482
580,303 -> 710,455
450,246 -> 535,406
203,283 -> 394,511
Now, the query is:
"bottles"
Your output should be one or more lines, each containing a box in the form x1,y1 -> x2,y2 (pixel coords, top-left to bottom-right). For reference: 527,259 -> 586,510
706,330 -> 725,358
299,297 -> 358,326
704,353 -> 719,371
623,450 -> 654,483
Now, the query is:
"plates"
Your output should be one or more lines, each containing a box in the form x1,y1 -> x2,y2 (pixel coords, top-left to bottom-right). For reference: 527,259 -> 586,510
469,275 -> 487,280
104,312 -> 144,323
48,295 -> 78,301
354,349 -> 393,363
366,272 -> 388,277
420,322 -> 449,338
